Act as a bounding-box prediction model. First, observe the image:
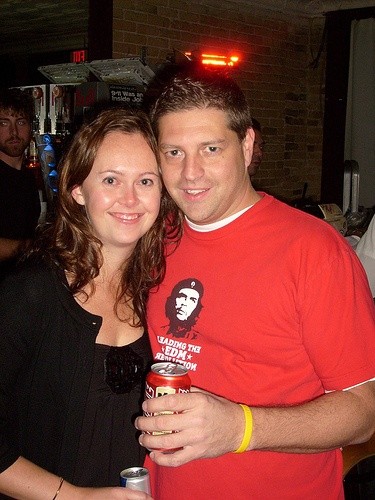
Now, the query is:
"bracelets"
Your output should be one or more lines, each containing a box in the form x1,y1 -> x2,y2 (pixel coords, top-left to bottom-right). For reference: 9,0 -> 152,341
53,477 -> 65,500
233,403 -> 254,455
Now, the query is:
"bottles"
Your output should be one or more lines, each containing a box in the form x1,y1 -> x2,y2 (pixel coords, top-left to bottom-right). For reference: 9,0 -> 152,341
37,201 -> 47,223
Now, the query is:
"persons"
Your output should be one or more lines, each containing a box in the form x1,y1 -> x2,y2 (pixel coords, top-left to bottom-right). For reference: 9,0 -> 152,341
0,108 -> 184,500
143,76 -> 375,500
0,87 -> 42,259
353,213 -> 375,299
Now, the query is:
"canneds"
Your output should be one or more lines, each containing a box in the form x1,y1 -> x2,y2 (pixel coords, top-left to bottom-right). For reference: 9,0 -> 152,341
120,467 -> 152,497
142,363 -> 191,453
